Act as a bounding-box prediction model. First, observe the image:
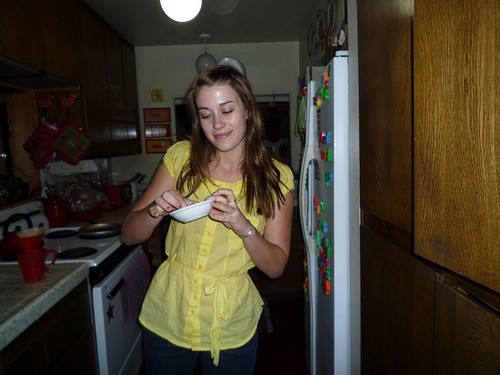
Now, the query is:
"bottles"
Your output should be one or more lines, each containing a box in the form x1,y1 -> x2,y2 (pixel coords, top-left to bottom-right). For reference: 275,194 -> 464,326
45,198 -> 67,228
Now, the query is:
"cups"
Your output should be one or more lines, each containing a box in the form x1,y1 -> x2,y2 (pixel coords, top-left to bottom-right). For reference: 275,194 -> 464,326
17,246 -> 57,284
11,229 -> 45,251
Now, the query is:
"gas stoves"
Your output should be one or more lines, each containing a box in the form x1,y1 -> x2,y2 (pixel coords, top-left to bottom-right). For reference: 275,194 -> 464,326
0,197 -> 123,267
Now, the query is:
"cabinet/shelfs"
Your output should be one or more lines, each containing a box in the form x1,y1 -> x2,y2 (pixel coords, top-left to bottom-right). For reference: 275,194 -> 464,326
0,278 -> 101,375
358,0 -> 500,374
0,0 -> 142,158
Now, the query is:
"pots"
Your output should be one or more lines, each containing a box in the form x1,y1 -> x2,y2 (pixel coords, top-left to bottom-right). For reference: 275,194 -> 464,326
100,172 -> 141,207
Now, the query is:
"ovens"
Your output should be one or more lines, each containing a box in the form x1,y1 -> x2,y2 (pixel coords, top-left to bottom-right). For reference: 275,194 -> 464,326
89,242 -> 152,375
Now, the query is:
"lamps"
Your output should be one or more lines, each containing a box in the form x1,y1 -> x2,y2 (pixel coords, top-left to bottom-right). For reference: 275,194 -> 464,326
196,34 -> 217,73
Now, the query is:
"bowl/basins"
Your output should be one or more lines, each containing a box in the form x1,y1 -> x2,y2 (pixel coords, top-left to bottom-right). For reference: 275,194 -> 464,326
70,203 -> 104,221
169,199 -> 216,223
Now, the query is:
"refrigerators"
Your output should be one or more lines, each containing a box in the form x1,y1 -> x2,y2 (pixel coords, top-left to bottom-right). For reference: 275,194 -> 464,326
299,51 -> 350,375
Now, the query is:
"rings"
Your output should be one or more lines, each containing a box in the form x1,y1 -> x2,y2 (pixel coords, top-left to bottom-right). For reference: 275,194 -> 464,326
227,200 -> 235,203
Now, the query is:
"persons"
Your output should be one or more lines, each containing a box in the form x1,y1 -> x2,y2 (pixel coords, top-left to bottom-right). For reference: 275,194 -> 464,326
122,66 -> 294,375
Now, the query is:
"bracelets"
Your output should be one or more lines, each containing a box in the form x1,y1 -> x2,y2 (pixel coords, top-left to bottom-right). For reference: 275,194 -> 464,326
236,221 -> 254,238
